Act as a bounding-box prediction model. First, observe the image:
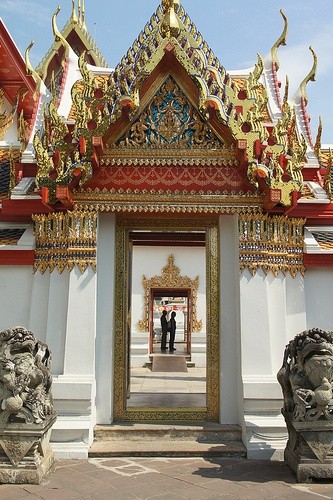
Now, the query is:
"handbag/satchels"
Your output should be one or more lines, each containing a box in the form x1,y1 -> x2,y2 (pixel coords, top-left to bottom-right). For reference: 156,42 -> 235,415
167,320 -> 176,331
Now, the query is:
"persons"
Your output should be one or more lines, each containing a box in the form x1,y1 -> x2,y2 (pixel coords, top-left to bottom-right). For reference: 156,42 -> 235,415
169,311 -> 176,352
161,309 -> 169,349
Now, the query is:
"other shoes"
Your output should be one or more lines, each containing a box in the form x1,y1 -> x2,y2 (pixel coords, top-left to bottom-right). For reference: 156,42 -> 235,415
161,347 -> 168,349
170,348 -> 176,351
